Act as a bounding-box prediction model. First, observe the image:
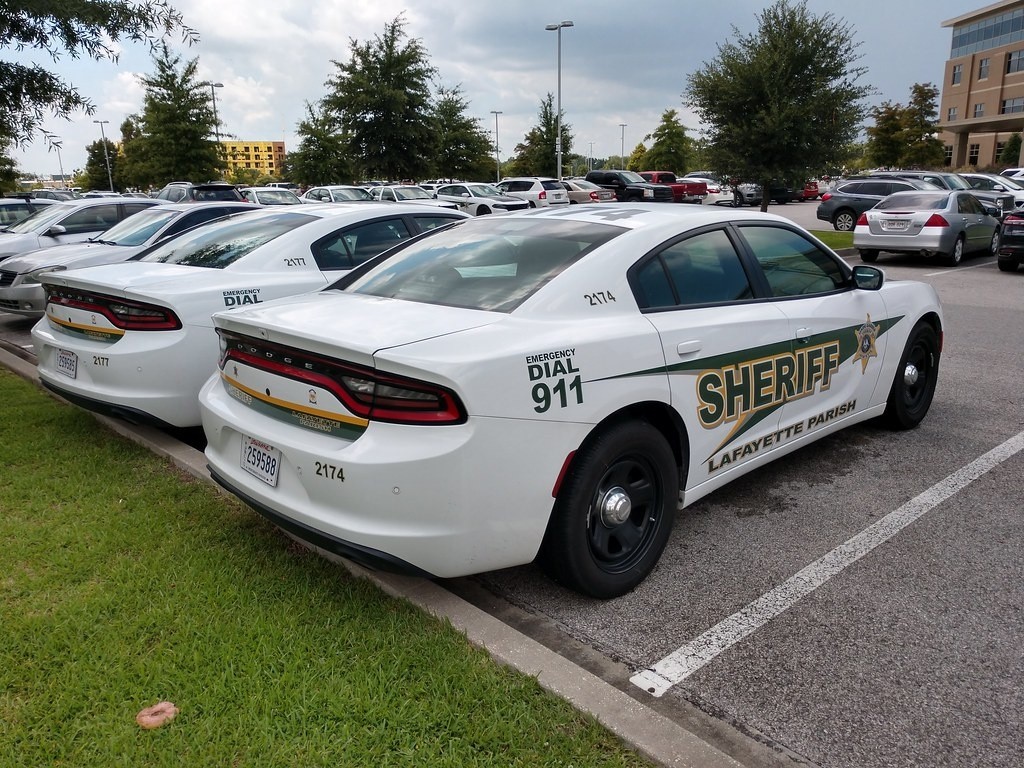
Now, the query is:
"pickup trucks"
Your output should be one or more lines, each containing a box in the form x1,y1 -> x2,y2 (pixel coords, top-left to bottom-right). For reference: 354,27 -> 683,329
636,171 -> 709,204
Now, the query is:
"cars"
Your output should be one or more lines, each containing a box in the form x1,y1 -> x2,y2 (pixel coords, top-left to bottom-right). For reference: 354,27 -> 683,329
675,177 -> 734,206
560,179 -> 617,205
771,169 -> 1024,224
240,186 -> 303,205
435,183 -> 531,217
995,202 -> 1024,272
30,199 -> 527,439
198,201 -> 943,601
298,185 -> 381,202
853,190 -> 1001,267
0,186 -> 402,321
361,185 -> 461,212
418,183 -> 448,199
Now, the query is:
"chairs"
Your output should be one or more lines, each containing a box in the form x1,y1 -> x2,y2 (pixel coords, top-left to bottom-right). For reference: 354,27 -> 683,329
353,223 -> 402,269
318,236 -> 347,268
516,234 -> 580,279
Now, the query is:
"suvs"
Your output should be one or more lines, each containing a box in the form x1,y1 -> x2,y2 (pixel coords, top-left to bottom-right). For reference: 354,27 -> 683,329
154,181 -> 250,204
493,177 -> 571,208
584,170 -> 676,202
685,171 -> 765,208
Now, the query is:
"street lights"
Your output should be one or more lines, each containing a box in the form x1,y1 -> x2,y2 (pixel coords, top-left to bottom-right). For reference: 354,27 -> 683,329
92,120 -> 114,192
545,20 -> 574,180
588,142 -> 595,172
490,110 -> 503,184
619,124 -> 627,170
201,80 -> 225,178
